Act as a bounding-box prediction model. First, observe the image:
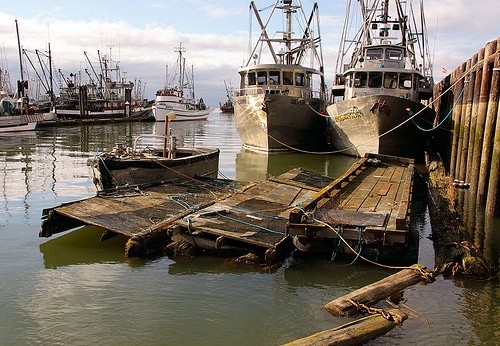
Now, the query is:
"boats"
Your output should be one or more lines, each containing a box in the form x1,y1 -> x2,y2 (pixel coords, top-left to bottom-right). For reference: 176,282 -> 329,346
87,115 -> 220,191
219,80 -> 234,112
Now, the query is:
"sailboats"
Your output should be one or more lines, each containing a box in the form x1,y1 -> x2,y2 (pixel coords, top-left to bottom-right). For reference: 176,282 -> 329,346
326,0 -> 437,164
151,41 -> 210,121
0,19 -> 154,132
231,0 -> 331,153
